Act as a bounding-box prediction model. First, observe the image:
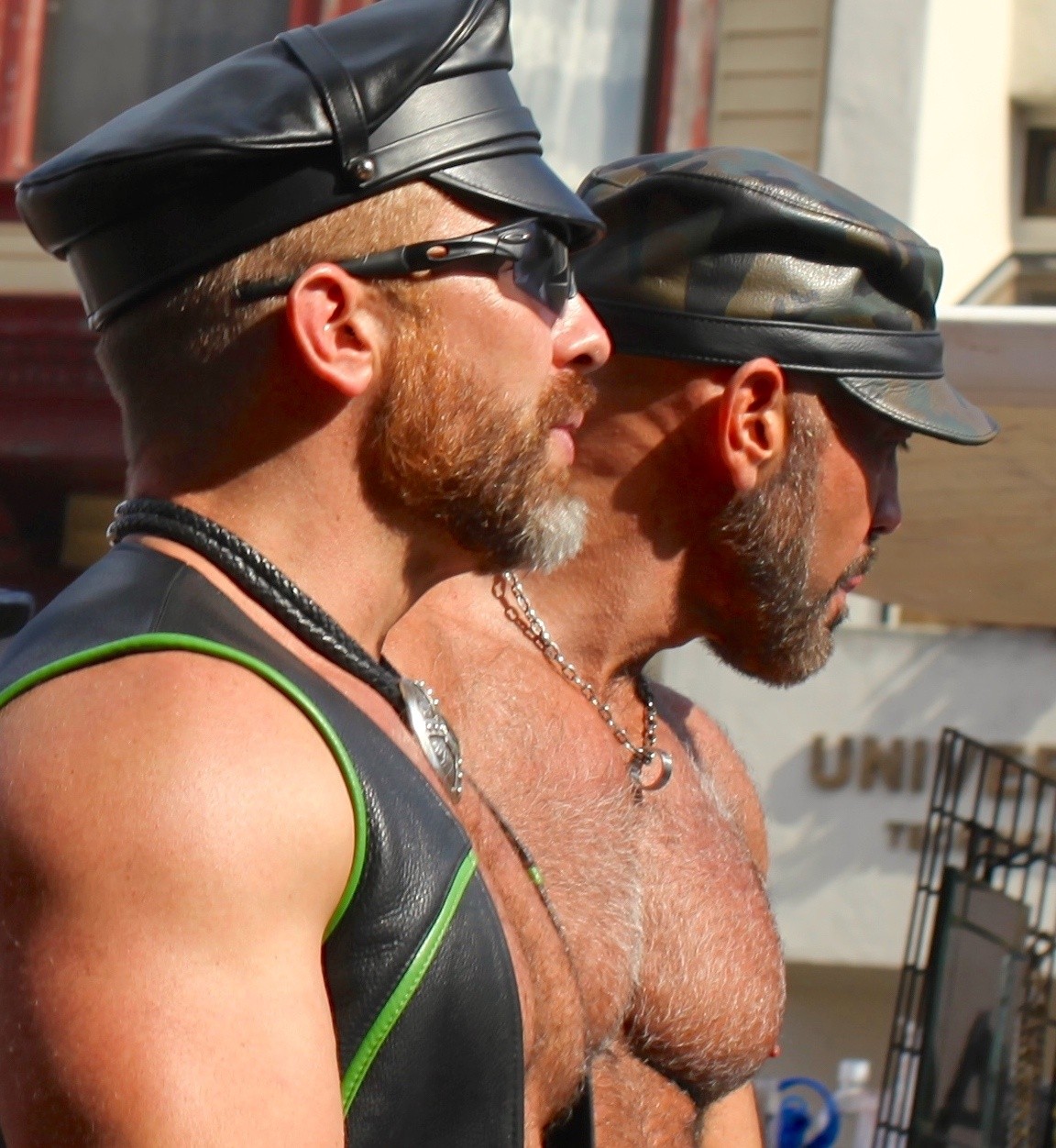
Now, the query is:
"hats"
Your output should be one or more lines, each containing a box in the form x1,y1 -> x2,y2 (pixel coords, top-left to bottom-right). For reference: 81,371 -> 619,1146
569,147 -> 999,446
13,0 -> 603,330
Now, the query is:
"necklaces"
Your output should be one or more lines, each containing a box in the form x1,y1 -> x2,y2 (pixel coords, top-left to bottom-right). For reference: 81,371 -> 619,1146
500,557 -> 674,793
108,492 -> 466,806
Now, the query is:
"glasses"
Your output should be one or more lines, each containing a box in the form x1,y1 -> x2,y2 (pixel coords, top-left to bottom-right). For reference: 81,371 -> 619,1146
228,212 -> 571,315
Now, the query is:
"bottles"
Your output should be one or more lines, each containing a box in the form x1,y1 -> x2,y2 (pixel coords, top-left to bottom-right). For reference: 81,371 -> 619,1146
819,1058 -> 875,1148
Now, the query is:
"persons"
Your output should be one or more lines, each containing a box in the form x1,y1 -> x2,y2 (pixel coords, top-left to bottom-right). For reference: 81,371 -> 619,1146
0,0 -> 999,1148
